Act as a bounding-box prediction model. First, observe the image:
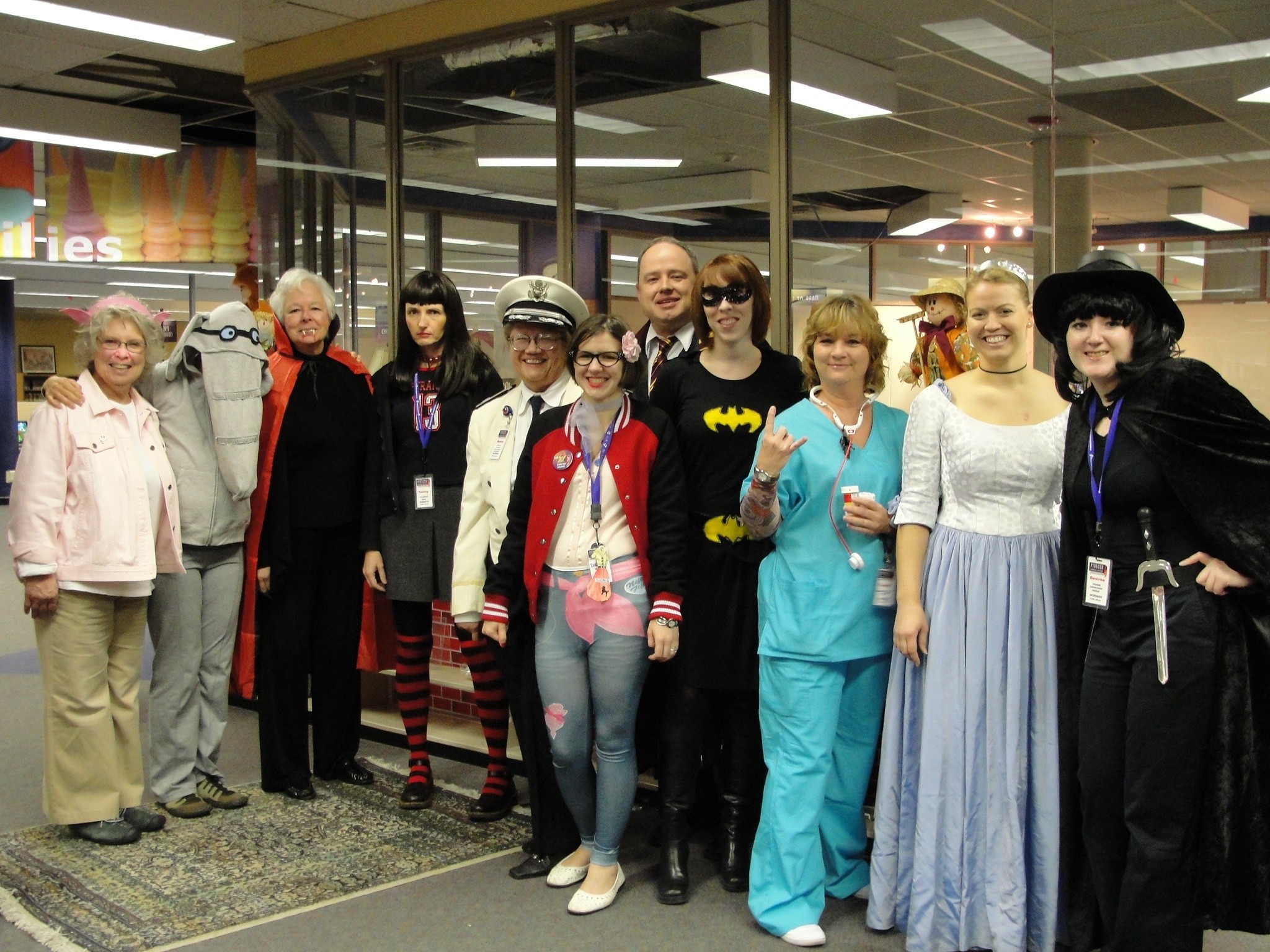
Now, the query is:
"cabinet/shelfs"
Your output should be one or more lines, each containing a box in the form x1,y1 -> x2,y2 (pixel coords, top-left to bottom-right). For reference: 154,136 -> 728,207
307,662 -> 660,793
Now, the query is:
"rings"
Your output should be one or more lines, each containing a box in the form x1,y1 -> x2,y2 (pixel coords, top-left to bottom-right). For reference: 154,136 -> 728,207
668,648 -> 675,654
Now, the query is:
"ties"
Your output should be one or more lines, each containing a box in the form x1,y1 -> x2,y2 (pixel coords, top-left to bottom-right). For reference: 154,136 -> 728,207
648,336 -> 679,393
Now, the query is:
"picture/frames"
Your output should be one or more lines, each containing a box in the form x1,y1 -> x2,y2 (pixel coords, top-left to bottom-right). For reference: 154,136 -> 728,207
19,345 -> 58,375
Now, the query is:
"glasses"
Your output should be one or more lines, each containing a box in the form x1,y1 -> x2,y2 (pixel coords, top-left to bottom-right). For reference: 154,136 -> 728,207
508,331 -> 561,351
568,350 -> 623,367
97,337 -> 147,355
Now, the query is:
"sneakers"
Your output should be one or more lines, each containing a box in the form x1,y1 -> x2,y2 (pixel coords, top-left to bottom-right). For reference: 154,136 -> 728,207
196,776 -> 248,809
157,793 -> 213,818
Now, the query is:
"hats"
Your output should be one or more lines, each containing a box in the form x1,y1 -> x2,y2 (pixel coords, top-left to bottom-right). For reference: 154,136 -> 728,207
494,275 -> 590,335
910,280 -> 966,310
1033,251 -> 1185,349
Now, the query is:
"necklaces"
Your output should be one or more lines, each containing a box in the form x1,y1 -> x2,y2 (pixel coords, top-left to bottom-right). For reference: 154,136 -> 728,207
975,359 -> 1027,374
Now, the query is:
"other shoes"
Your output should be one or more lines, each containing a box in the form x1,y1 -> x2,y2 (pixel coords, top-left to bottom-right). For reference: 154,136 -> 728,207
119,807 -> 166,832
782,924 -> 827,946
546,851 -> 589,887
567,861 -> 626,915
399,769 -> 434,809
68,818 -> 141,846
338,759 -> 374,784
468,771 -> 517,820
285,780 -> 316,800
854,884 -> 871,900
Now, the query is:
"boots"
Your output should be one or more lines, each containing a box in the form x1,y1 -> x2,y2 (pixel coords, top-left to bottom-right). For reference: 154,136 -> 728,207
719,791 -> 749,891
657,804 -> 689,906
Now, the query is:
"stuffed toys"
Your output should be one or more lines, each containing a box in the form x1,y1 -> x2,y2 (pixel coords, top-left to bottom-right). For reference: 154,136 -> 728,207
899,275 -> 980,389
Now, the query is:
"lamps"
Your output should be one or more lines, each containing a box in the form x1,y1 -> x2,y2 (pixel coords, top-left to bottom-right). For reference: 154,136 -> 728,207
700,20 -> 897,120
1166,186 -> 1250,232
886,192 -> 963,238
0,85 -> 181,158
615,170 -> 771,215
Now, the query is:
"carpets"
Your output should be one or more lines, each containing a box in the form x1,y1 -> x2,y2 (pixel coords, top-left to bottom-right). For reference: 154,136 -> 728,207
0,756 -> 533,952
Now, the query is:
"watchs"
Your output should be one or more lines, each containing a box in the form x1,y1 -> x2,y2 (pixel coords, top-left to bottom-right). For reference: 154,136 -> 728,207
655,615 -> 679,629
751,462 -> 779,485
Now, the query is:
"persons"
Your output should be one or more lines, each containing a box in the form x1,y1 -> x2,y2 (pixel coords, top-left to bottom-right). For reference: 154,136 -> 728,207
866,265 -> 1086,952
444,276 -> 591,880
479,311 -> 691,917
241,266 -> 376,801
6,286 -> 169,852
361,272 -> 515,827
40,301 -> 274,822
739,296 -> 907,949
645,252 -> 803,908
1031,259 -> 1270,952
624,237 -> 708,852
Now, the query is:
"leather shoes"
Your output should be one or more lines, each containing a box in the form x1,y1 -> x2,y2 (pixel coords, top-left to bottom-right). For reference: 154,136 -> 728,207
509,839 -> 553,880
